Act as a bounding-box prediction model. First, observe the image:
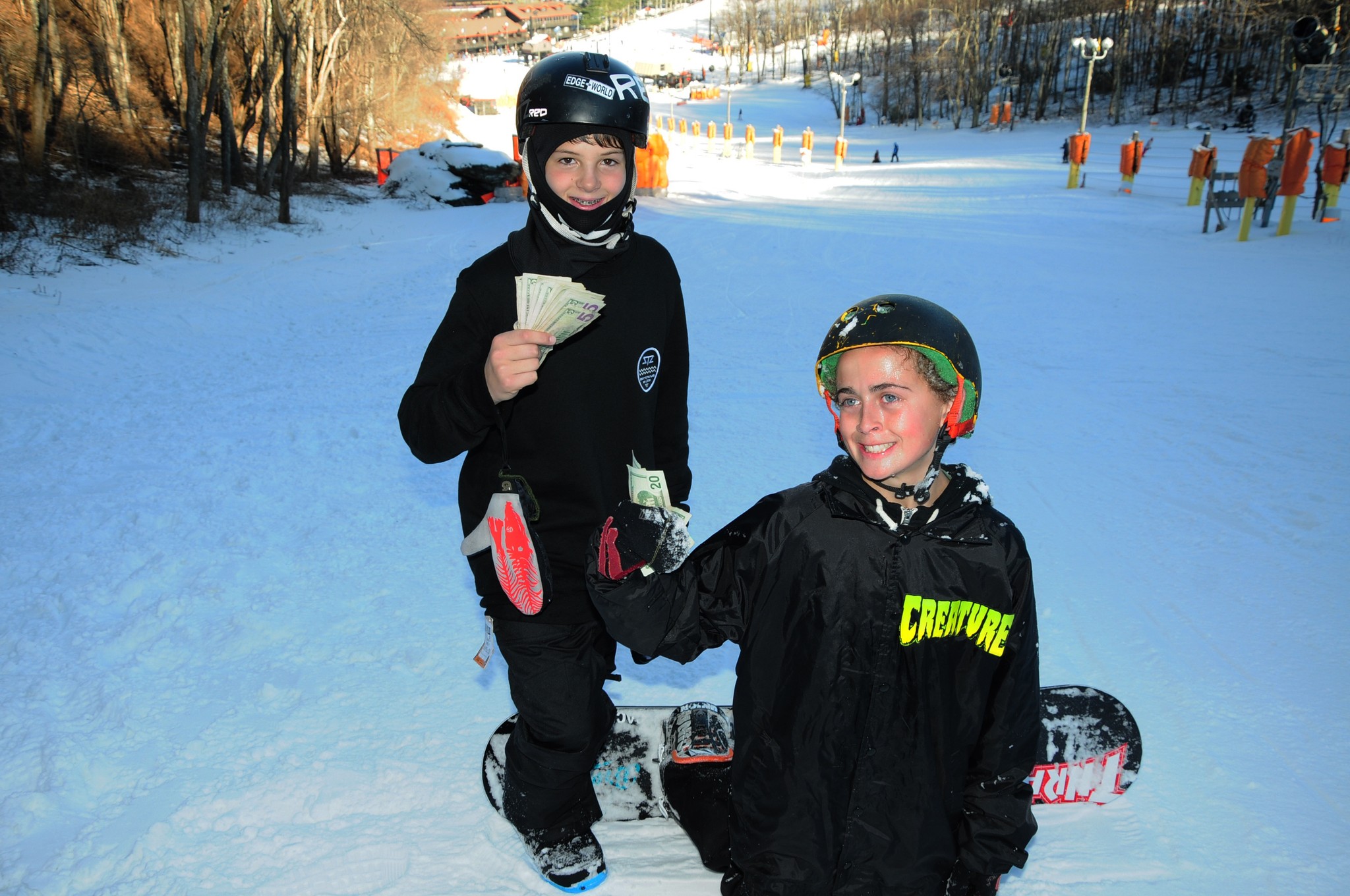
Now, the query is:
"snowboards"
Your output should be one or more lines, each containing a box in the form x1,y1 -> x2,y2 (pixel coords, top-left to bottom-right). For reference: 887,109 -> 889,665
482,685 -> 1143,823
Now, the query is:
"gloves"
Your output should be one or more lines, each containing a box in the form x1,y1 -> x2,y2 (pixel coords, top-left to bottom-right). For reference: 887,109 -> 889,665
650,515 -> 692,574
593,501 -> 672,580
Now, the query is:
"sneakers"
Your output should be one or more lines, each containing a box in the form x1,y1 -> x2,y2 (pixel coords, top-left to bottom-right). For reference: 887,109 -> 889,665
509,817 -> 608,895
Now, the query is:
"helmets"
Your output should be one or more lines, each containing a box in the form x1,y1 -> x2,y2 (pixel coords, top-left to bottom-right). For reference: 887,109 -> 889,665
515,51 -> 649,148
814,294 -> 981,440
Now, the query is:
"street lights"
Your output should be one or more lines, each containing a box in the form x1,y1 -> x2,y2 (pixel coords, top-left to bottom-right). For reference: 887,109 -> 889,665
1071,35 -> 1114,133
828,71 -> 862,139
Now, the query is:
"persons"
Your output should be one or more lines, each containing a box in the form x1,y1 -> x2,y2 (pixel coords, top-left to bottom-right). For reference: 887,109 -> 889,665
397,50 -> 694,893
1143,138 -> 1154,156
872,150 -> 881,163
890,143 -> 899,162
1060,138 -> 1069,163
585,294 -> 1043,896
